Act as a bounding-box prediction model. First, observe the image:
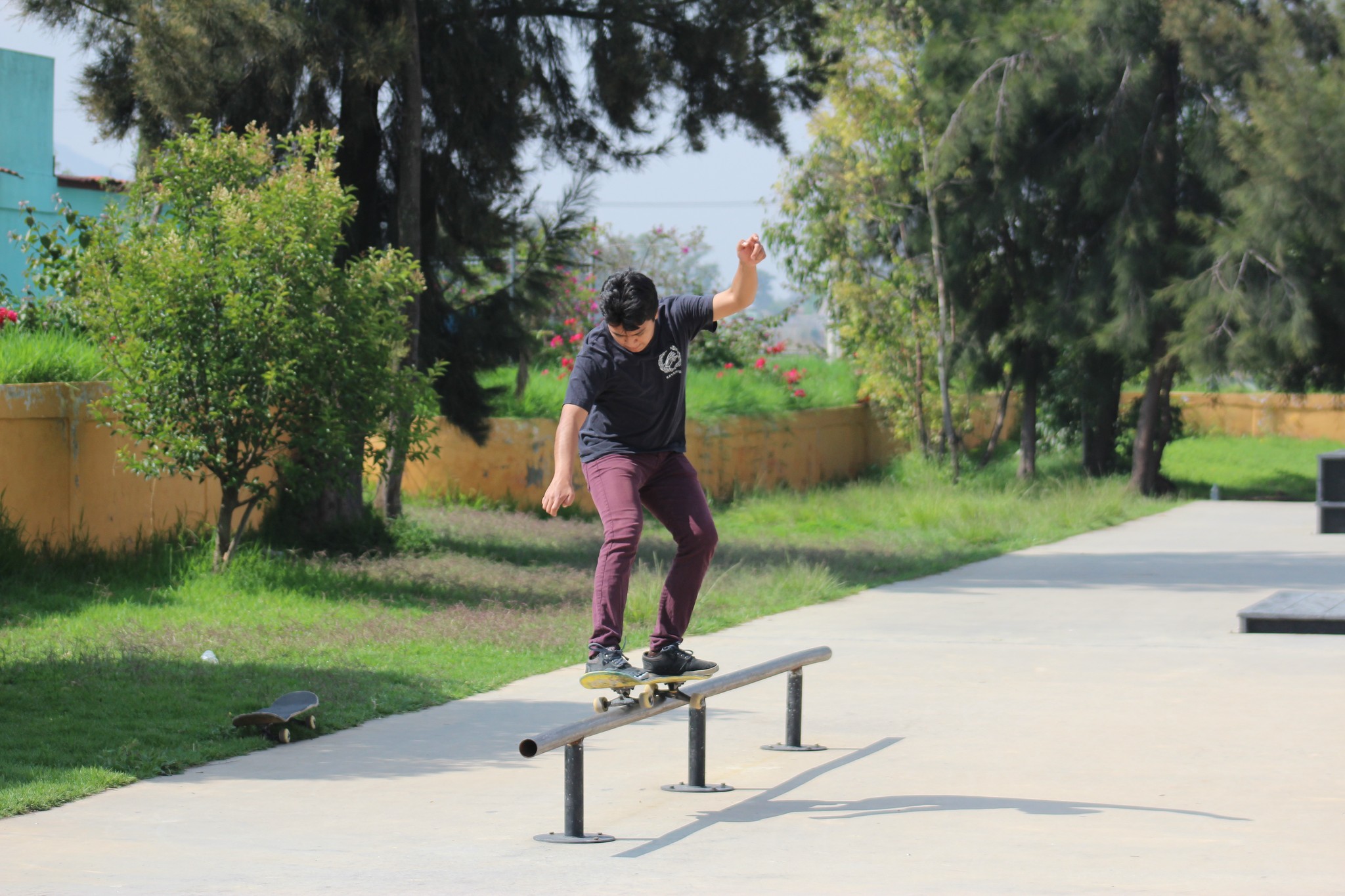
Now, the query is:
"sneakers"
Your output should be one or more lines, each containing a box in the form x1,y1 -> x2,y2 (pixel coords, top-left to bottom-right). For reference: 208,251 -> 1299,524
642,641 -> 720,677
584,636 -> 650,680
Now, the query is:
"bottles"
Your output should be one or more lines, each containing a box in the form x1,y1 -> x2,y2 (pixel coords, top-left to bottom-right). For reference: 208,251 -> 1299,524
1210,484 -> 1219,500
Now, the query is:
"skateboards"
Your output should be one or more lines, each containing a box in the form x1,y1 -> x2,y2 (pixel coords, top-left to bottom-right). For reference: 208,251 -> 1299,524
580,671 -> 713,714
232,690 -> 320,743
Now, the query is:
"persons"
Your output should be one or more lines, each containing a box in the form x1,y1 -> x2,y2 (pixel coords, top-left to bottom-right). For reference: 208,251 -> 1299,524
541,232 -> 767,681
